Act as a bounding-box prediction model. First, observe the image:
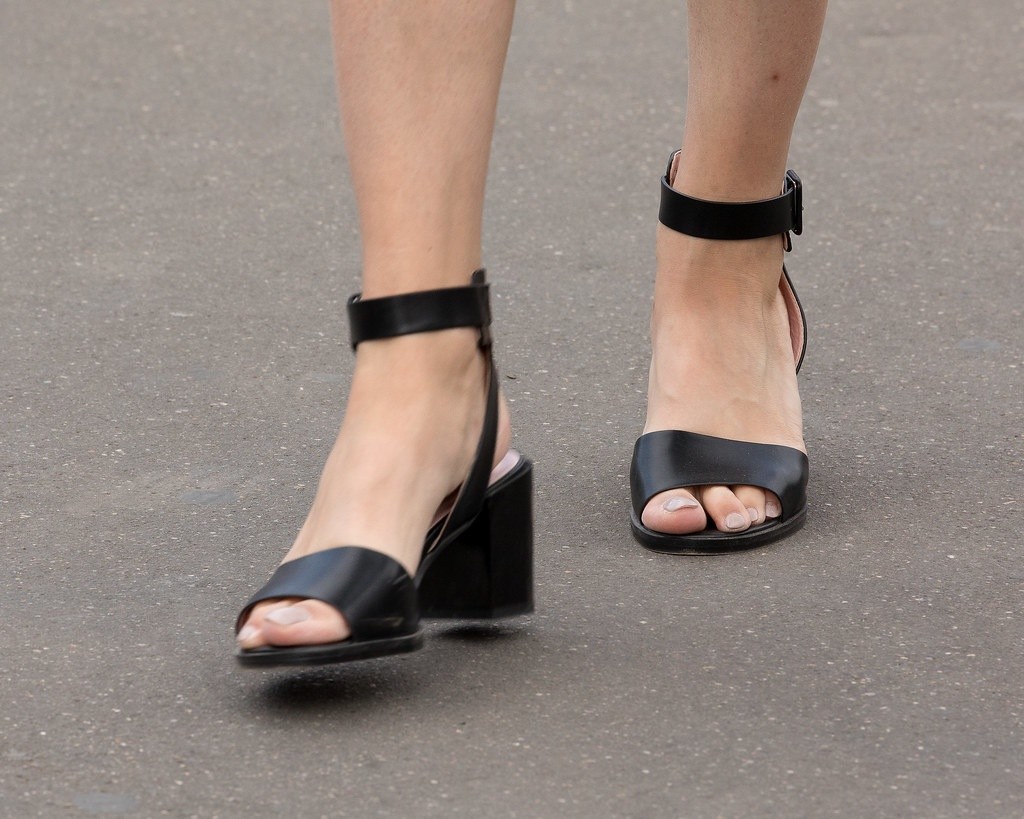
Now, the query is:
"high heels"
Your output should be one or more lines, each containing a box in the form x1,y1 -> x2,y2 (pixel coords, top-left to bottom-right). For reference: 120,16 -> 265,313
629,149 -> 809,556
234,267 -> 533,667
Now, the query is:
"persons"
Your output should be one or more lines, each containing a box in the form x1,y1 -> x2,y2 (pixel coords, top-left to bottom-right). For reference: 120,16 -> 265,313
236,0 -> 832,666
